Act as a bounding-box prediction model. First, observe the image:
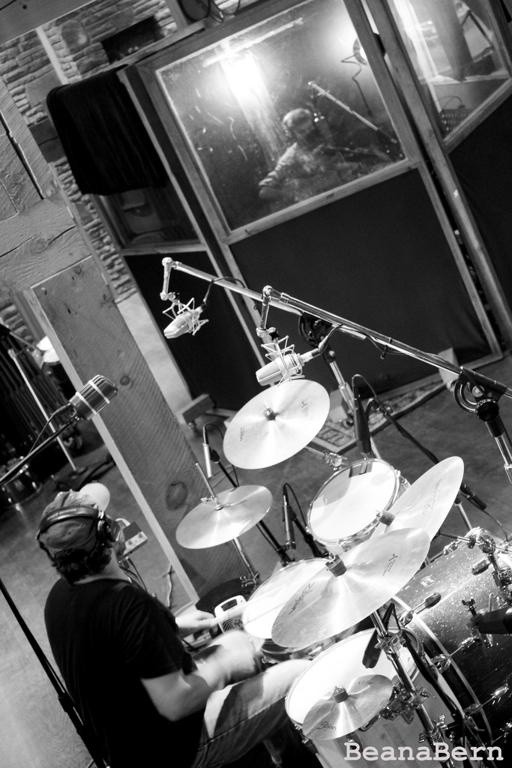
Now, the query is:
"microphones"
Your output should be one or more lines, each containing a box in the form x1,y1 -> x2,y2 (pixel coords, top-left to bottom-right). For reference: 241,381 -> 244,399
255,336 -> 312,387
162,301 -> 208,340
67,374 -> 118,422
314,114 -> 331,136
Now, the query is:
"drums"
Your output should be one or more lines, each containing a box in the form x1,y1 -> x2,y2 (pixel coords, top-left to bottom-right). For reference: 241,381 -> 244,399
285,627 -> 467,767
0,462 -> 45,508
377,527 -> 510,766
242,557 -> 356,663
303,458 -> 411,553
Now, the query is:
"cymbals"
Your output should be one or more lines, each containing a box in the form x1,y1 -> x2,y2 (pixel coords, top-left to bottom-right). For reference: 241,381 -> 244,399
175,485 -> 272,550
303,675 -> 393,740
223,379 -> 330,470
369,456 -> 464,544
272,529 -> 431,649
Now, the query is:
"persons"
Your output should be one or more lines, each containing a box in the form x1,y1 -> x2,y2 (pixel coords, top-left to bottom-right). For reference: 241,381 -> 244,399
258,108 -> 359,202
36,482 -> 312,767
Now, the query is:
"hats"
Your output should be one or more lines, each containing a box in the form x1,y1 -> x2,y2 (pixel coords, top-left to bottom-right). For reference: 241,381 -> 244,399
38,482 -> 111,561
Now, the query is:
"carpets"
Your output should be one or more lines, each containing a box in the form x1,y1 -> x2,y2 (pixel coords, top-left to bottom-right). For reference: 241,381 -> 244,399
305,380 -> 444,456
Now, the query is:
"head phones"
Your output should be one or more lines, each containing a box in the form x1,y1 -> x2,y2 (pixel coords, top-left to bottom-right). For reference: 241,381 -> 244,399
281,109 -> 313,146
35,507 -> 121,546
